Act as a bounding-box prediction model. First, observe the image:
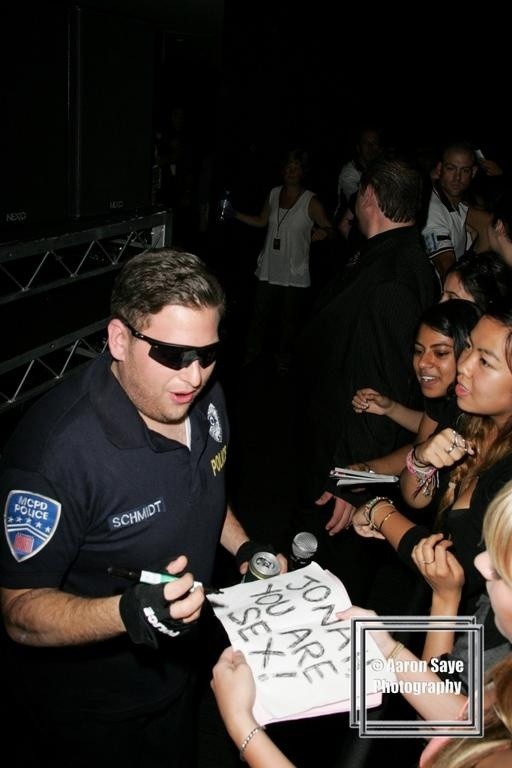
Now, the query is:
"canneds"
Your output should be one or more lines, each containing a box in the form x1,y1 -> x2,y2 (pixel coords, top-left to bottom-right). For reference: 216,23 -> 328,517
241,551 -> 281,583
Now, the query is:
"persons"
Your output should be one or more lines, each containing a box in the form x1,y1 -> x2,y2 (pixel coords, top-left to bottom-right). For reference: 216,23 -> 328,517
0,248 -> 290,767
209,120 -> 511,768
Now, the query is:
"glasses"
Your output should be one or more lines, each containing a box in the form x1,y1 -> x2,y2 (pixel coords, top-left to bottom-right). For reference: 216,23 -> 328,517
106,313 -> 221,369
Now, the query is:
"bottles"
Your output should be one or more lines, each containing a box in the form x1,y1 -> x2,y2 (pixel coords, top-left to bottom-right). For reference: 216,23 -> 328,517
216,190 -> 233,223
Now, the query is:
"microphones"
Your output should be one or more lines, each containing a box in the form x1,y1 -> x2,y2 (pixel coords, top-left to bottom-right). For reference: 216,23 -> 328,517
288,531 -> 318,572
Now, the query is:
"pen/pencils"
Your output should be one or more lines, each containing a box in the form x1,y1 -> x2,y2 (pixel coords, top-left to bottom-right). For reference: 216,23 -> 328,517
108,565 -> 225,595
